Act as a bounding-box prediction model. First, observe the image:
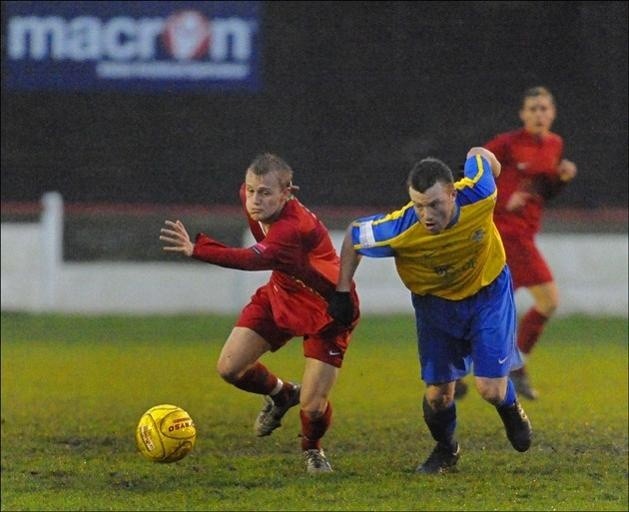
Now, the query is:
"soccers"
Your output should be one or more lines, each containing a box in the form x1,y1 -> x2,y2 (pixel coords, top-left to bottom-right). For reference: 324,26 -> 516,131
135,404 -> 195,462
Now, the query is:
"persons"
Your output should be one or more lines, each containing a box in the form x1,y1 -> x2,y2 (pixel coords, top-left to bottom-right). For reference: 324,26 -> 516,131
328,145 -> 531,476
159,154 -> 359,474
483,87 -> 576,401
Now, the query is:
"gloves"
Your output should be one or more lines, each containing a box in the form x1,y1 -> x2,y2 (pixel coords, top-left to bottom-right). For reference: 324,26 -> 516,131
326,291 -> 354,326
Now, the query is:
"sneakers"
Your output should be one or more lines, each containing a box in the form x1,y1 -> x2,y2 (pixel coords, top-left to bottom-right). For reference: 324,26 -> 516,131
255,382 -> 303,437
496,393 -> 533,452
301,447 -> 334,477
415,439 -> 462,478
510,366 -> 539,400
